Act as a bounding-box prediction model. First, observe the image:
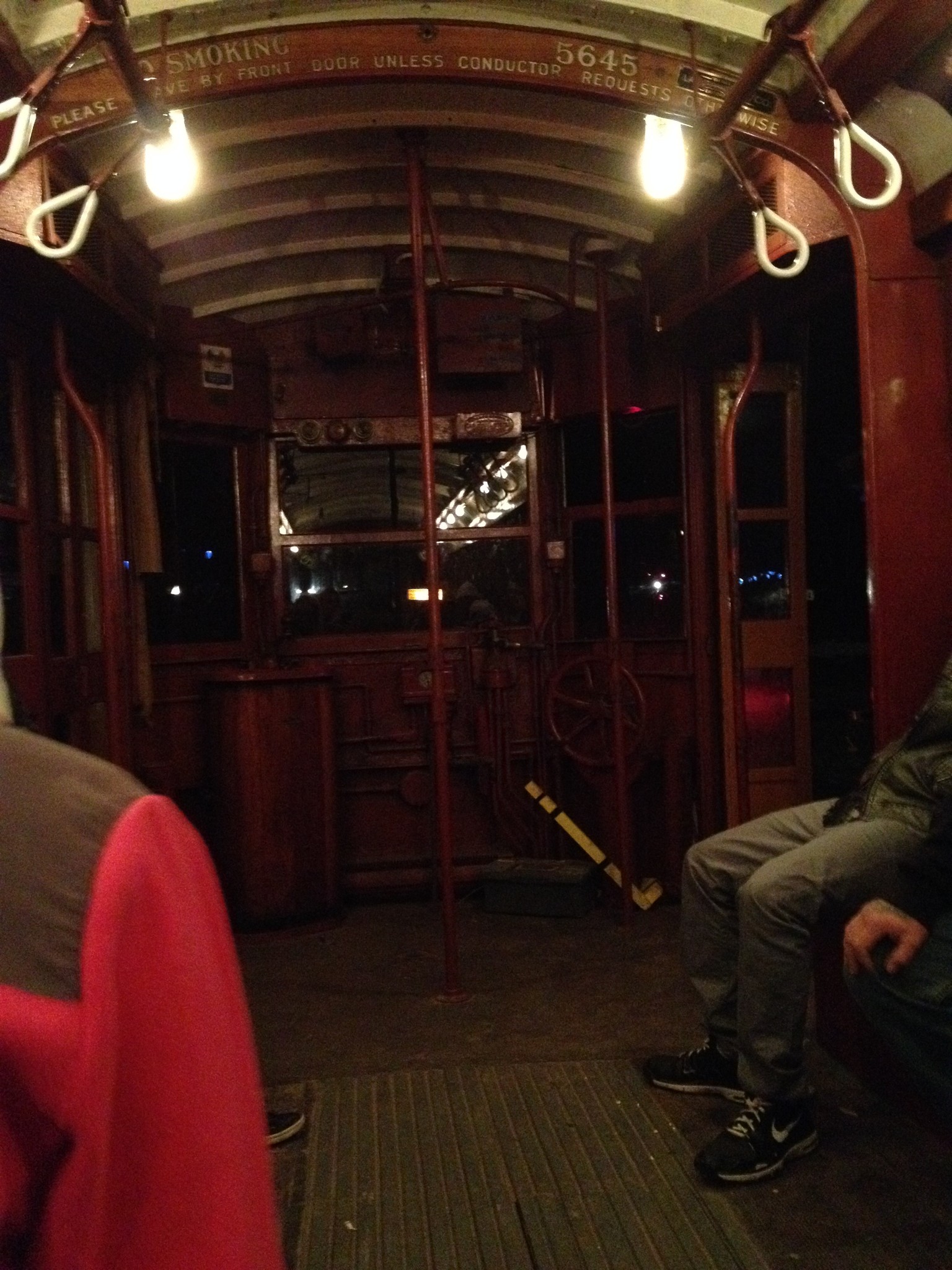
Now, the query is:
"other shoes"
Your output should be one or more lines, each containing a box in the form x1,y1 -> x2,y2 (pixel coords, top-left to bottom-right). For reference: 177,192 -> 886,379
266,1112 -> 308,1143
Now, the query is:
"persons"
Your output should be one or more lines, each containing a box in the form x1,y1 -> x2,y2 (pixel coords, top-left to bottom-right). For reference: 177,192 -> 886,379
263,1106 -> 305,1145
642,651 -> 951,1190
3,680 -> 303,1270
851,765 -> 952,1128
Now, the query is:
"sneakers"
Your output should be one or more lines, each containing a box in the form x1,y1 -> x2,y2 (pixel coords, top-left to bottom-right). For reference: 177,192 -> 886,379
696,1092 -> 824,1186
643,1037 -> 750,1104
